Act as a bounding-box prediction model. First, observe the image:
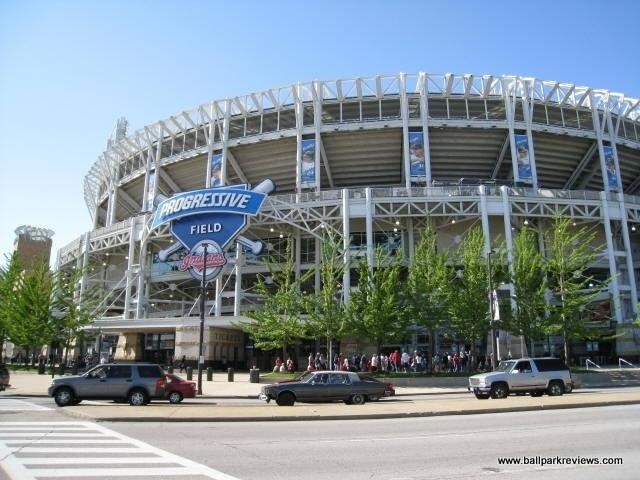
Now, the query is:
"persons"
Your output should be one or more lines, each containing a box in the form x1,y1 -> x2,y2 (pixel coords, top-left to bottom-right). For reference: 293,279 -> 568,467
302,150 -> 314,171
606,158 -> 616,174
271,350 -> 495,373
410,144 -> 424,168
518,147 -> 529,163
0,363 -> 10,392
209,162 -> 221,187
179,355 -> 188,374
37,351 -> 99,370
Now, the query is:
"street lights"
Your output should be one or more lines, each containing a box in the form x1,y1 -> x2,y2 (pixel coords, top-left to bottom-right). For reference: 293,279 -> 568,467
487,248 -> 498,370
97,327 -> 104,363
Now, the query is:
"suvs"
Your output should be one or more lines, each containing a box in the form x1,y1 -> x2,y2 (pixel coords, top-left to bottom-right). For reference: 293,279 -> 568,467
49,362 -> 168,406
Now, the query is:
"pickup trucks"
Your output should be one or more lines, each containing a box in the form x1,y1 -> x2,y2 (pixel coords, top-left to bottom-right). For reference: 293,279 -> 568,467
467,358 -> 573,400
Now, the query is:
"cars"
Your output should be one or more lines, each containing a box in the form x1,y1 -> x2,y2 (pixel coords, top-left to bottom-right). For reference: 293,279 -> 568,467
259,370 -> 396,407
0,362 -> 10,395
162,375 -> 199,403
